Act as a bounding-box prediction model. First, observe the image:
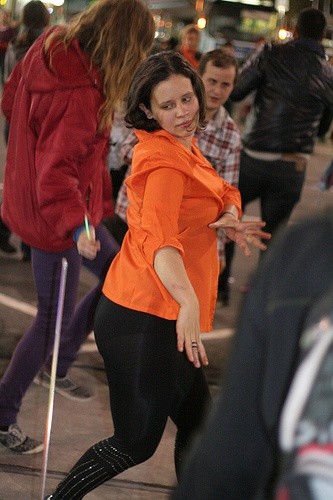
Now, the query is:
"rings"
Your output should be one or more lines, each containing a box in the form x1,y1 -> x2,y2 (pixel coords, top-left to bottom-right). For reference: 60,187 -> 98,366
191,342 -> 199,350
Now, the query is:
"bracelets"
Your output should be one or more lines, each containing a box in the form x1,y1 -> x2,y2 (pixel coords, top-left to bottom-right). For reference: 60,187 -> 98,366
222,210 -> 235,217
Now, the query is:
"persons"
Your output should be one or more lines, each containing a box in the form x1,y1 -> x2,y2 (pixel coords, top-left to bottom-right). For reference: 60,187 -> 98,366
86,25 -> 268,343
42,50 -> 271,500
215,8 -> 332,310
3,0 -> 156,457
173,184 -> 333,500
0,0 -> 52,264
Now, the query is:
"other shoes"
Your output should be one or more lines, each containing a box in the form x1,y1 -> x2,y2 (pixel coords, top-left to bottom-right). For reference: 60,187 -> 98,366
0,424 -> 45,456
33,369 -> 96,403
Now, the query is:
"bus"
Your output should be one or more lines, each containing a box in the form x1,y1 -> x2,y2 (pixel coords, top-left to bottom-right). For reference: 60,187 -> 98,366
193,0 -> 280,66
